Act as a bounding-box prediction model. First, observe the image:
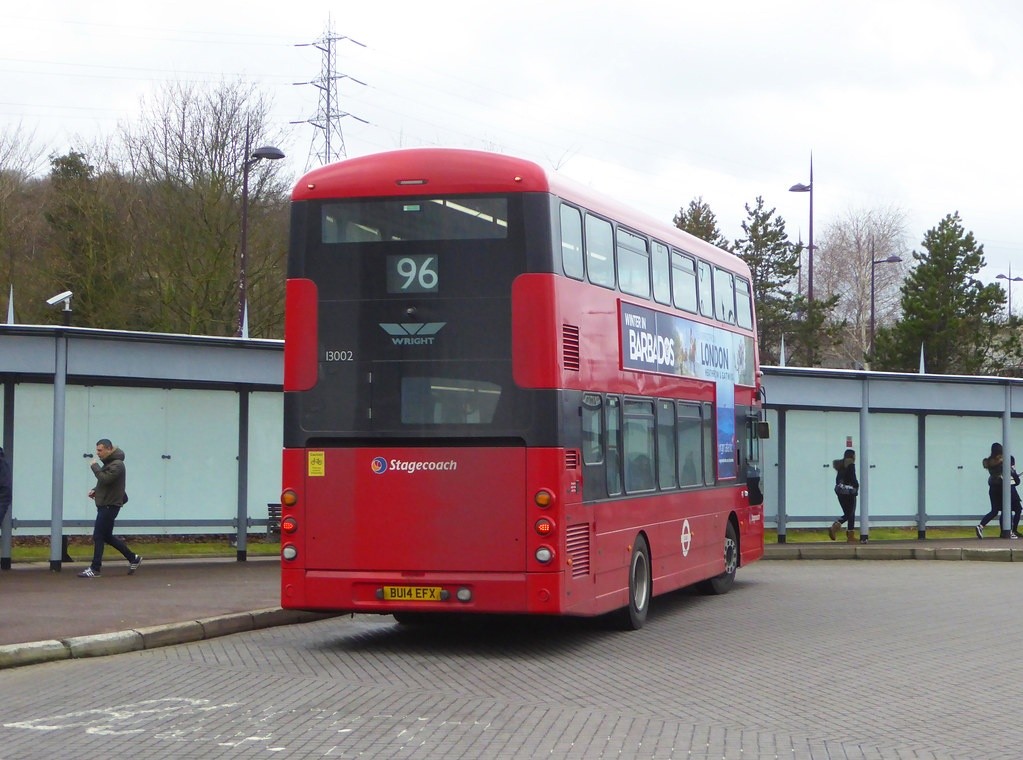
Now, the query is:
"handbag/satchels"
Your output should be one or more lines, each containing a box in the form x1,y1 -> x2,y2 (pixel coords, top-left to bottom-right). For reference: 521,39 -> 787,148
1001,475 -> 1016,487
122,492 -> 128,506
834,478 -> 859,497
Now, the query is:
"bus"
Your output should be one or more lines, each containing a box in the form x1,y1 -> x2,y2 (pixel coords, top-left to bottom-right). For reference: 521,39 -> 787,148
276,147 -> 771,635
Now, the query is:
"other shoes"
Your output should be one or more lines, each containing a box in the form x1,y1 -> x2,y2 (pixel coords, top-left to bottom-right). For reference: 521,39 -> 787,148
1013,531 -> 1023,539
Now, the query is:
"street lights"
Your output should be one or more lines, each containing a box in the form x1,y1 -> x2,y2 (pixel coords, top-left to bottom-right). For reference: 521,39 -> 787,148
789,149 -> 813,367
996,263 -> 1023,324
869,234 -> 903,370
237,112 -> 285,338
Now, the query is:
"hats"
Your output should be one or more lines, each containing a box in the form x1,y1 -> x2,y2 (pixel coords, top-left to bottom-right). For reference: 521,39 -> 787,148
990,443 -> 1004,457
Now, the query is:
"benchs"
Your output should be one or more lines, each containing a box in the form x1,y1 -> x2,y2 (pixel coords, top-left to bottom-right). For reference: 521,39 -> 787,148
265,502 -> 282,542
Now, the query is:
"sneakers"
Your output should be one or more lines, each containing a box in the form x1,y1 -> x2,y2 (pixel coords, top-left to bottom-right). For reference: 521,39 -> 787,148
975,524 -> 984,539
127,553 -> 143,573
77,568 -> 102,578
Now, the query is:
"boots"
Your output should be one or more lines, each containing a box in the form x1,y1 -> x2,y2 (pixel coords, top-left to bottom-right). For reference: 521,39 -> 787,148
829,521 -> 841,539
846,529 -> 861,542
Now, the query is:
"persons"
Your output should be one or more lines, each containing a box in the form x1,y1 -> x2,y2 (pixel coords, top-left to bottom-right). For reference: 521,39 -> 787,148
976,442 -> 1022,539
826,449 -> 864,543
75,439 -> 145,578
